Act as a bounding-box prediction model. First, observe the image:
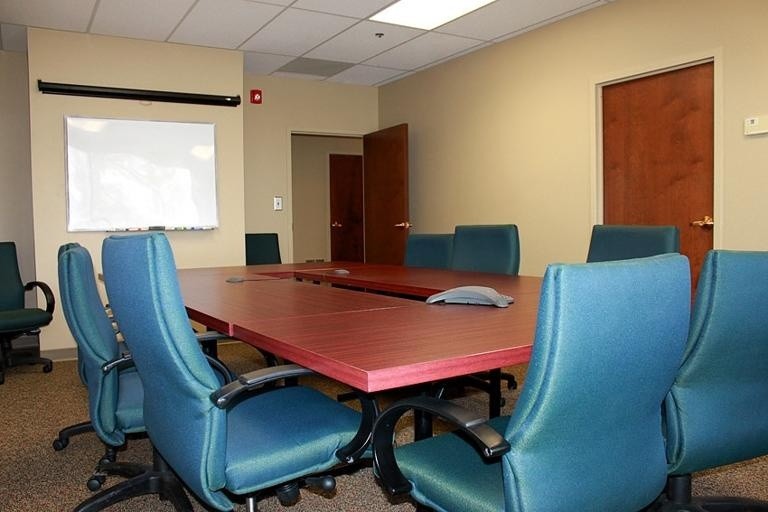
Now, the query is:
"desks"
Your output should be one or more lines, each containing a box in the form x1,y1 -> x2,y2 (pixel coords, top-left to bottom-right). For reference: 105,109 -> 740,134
287,268 -> 552,417
235,293 -> 554,512
177,279 -> 428,387
178,260 -> 398,357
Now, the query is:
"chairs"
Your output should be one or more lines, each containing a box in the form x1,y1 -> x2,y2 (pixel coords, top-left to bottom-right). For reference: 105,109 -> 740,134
428,225 -> 520,407
246,234 -> 282,264
58,241 -> 199,386
74,232 -> 378,512
0,242 -> 56,386
373,251 -> 692,512
402,234 -> 452,271
53,250 -> 277,512
643,249 -> 768,511
587,224 -> 677,261
